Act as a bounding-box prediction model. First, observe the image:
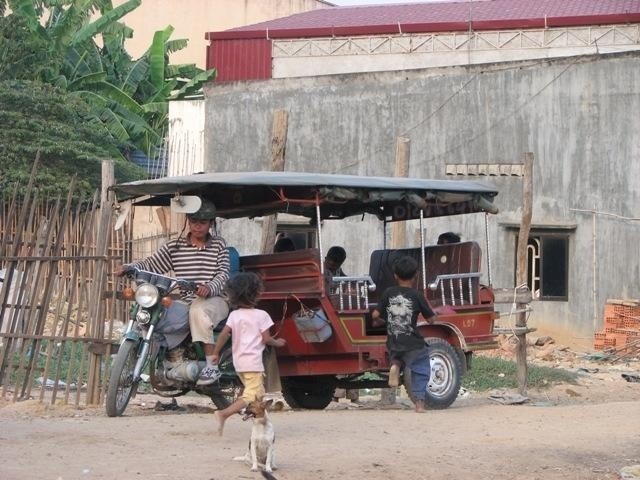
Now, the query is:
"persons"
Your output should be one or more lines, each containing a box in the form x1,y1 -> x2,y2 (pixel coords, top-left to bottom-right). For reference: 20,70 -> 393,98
273,237 -> 296,253
436,231 -> 461,245
324,245 -> 385,328
112,198 -> 232,386
207,270 -> 286,439
372,255 -> 436,414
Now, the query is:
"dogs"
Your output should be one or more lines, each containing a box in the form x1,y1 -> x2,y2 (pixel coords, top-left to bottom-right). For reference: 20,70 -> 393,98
232,395 -> 278,473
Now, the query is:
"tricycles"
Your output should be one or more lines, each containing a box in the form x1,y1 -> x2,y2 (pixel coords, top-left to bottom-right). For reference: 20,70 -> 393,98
98,169 -> 504,412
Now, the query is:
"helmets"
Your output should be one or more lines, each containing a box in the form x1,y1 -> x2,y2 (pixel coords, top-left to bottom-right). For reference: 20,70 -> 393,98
187,198 -> 217,220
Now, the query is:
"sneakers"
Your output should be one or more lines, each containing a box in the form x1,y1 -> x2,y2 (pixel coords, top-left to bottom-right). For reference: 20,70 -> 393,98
196,365 -> 221,386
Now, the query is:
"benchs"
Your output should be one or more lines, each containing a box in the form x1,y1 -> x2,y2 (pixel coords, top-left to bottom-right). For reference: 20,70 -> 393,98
237,241 -> 482,316
